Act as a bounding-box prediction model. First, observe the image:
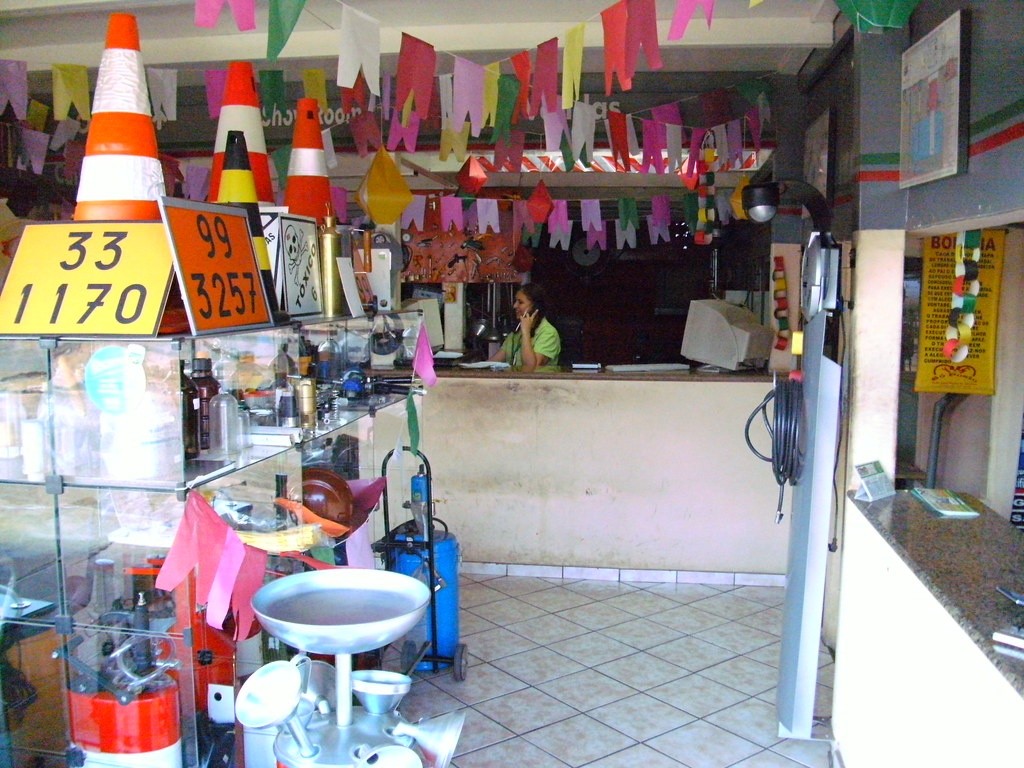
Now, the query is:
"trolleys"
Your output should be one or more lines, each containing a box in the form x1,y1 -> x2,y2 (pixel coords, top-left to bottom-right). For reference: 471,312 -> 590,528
371,446 -> 469,683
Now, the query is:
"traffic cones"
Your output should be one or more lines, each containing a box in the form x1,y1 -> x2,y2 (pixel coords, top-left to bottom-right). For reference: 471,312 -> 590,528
216,128 -> 291,324
72,13 -> 189,335
281,96 -> 335,224
204,60 -> 277,207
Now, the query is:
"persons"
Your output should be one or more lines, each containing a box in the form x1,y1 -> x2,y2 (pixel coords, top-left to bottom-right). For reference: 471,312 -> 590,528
485,285 -> 561,371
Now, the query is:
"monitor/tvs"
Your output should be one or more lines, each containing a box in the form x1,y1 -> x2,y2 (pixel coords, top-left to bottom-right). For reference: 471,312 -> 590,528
400,298 -> 444,353
681,299 -> 774,373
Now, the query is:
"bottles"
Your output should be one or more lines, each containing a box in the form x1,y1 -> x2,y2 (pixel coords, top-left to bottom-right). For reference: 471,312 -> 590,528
316,217 -> 344,317
270,343 -> 296,384
164,359 -> 201,460
209,387 -> 238,453
189,358 -> 220,449
318,331 -> 340,372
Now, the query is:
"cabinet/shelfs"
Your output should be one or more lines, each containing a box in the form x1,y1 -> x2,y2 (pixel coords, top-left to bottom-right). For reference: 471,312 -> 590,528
0,308 -> 424,768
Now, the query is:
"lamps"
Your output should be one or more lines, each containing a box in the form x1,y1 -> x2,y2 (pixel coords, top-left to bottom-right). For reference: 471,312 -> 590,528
742,184 -> 780,222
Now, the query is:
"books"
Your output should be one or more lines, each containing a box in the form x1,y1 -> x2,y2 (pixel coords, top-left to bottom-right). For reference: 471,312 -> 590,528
570,360 -> 598,370
994,643 -> 1024,661
991,627 -> 1024,651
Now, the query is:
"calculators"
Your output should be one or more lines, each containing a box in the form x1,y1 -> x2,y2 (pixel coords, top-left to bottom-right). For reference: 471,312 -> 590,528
996,586 -> 1024,607
